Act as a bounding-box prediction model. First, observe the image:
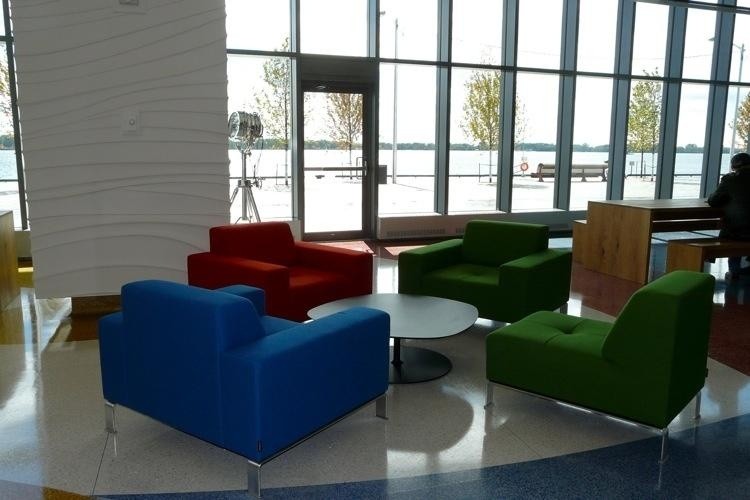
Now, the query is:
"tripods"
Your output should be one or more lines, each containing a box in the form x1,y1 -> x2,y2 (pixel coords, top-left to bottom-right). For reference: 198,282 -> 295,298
230,149 -> 261,224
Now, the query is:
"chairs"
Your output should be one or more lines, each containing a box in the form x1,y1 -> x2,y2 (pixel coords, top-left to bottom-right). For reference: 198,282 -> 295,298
396,220 -> 573,324
485,270 -> 717,464
96,279 -> 390,497
186,221 -> 374,322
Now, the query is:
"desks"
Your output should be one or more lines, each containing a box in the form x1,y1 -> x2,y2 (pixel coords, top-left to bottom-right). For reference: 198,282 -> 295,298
587,199 -> 720,283
306,292 -> 479,385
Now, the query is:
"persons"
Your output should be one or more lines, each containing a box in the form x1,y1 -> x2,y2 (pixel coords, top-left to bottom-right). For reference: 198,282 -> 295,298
708,153 -> 750,279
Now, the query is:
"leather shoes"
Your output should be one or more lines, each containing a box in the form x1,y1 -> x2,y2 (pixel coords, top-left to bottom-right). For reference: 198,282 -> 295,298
725,272 -> 739,281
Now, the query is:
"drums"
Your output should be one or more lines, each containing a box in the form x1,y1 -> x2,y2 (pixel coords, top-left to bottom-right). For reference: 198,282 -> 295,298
228,112 -> 263,143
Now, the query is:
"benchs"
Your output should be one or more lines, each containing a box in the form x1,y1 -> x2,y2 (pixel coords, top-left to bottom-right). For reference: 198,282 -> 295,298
531,163 -> 608,182
572,219 -> 721,257
665,238 -> 749,276
